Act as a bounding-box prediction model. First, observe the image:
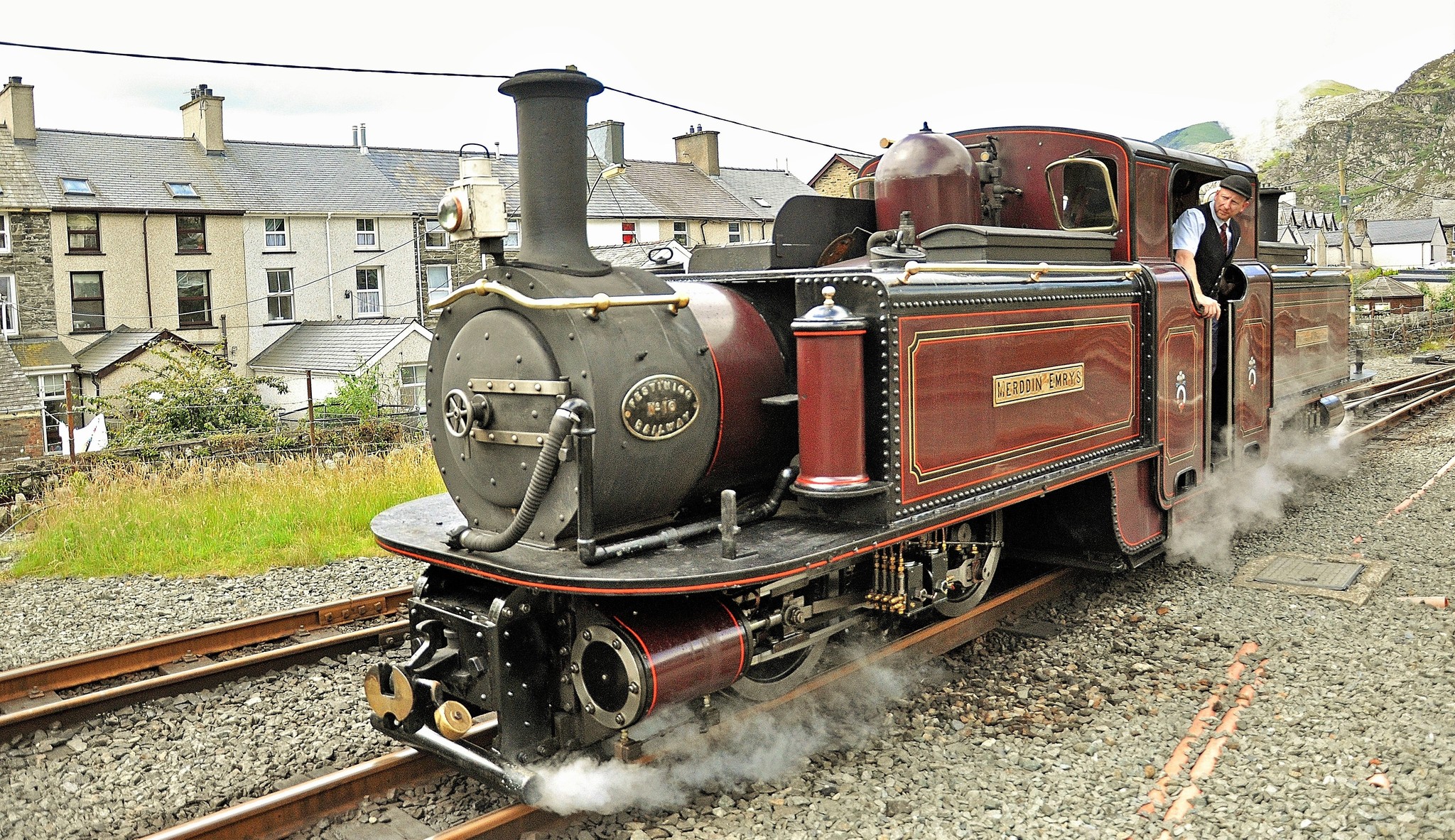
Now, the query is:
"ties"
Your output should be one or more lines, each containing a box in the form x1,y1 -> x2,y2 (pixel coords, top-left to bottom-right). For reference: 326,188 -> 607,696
1219,223 -> 1228,257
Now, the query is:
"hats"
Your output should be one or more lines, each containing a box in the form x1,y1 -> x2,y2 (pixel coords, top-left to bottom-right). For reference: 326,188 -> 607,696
1219,175 -> 1253,200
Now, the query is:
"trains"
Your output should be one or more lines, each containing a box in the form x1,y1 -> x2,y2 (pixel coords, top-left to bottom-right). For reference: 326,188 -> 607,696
364,64 -> 1362,807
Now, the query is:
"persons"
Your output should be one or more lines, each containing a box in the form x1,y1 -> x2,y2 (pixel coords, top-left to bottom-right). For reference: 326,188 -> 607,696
1172,175 -> 1253,456
